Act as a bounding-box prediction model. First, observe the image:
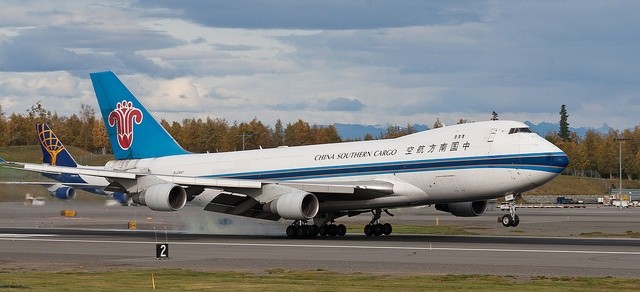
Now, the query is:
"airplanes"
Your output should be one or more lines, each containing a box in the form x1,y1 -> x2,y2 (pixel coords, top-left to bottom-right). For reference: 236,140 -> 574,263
0,67 -> 569,240
